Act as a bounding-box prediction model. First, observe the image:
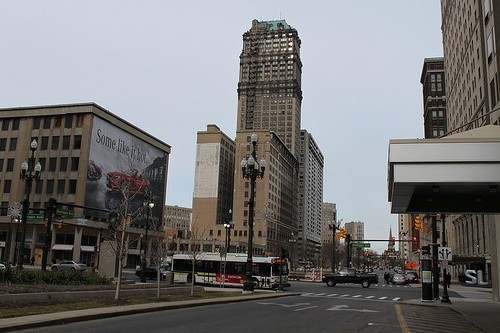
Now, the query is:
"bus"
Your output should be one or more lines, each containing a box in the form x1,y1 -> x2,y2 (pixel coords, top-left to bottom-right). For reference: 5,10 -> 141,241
170,251 -> 290,290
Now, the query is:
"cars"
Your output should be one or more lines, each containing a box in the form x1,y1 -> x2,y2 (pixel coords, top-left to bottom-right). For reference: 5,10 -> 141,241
391,266 -> 419,285
135,266 -> 172,280
0,262 -> 15,269
51,260 -> 88,273
104,168 -> 152,195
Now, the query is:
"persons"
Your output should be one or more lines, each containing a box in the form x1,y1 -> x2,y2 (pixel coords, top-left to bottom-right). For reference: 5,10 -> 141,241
384,272 -> 390,284
446,272 -> 451,288
12,259 -> 17,266
31,255 -> 35,266
51,256 -> 56,264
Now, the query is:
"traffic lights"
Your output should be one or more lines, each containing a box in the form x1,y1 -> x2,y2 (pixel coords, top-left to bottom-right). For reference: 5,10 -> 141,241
392,237 -> 396,245
339,227 -> 346,239
414,215 -> 421,232
412,236 -> 417,246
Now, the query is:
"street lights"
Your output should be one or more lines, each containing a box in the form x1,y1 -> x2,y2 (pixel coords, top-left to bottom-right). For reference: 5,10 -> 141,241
139,191 -> 155,283
223,208 -> 232,253
241,132 -> 266,291
327,208 -> 341,273
17,138 -> 42,270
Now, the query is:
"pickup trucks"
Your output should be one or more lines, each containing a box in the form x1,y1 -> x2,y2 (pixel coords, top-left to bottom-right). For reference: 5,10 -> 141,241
322,268 -> 378,288
